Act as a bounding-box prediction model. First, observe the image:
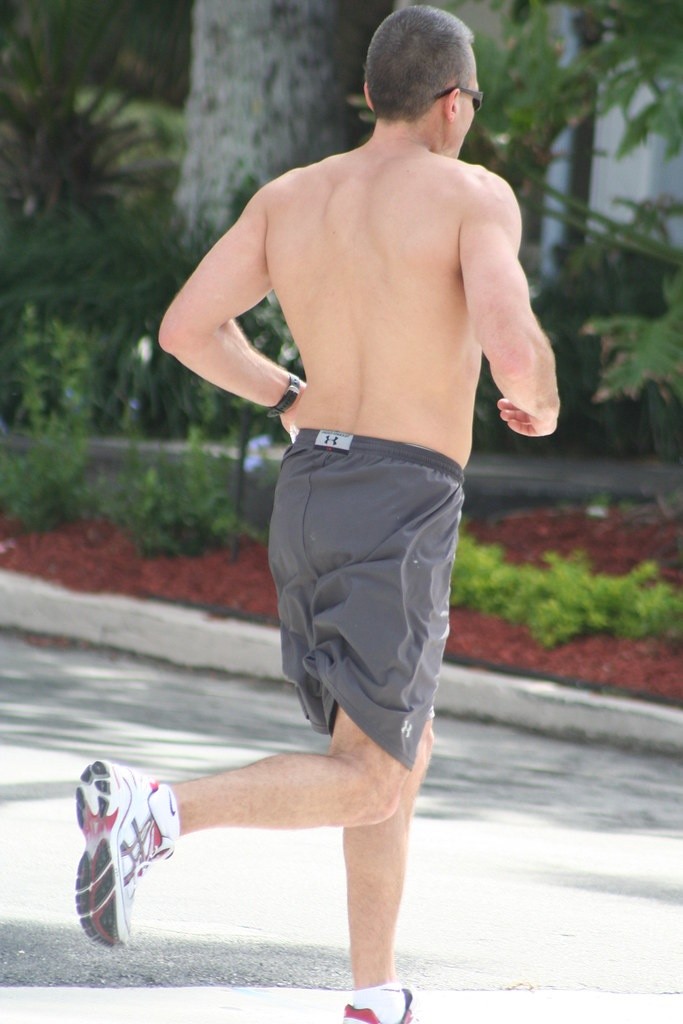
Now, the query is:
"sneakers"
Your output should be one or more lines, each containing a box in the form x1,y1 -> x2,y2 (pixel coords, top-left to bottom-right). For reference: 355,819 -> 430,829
73,757 -> 171,950
341,989 -> 420,1023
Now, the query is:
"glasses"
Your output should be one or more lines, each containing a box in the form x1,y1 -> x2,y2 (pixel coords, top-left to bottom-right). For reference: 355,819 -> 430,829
444,82 -> 488,112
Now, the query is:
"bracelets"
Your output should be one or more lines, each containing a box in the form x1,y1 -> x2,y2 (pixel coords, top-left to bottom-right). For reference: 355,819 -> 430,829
266,371 -> 301,418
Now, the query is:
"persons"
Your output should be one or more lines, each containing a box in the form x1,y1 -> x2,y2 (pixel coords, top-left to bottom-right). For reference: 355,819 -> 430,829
73,5 -> 566,1024
